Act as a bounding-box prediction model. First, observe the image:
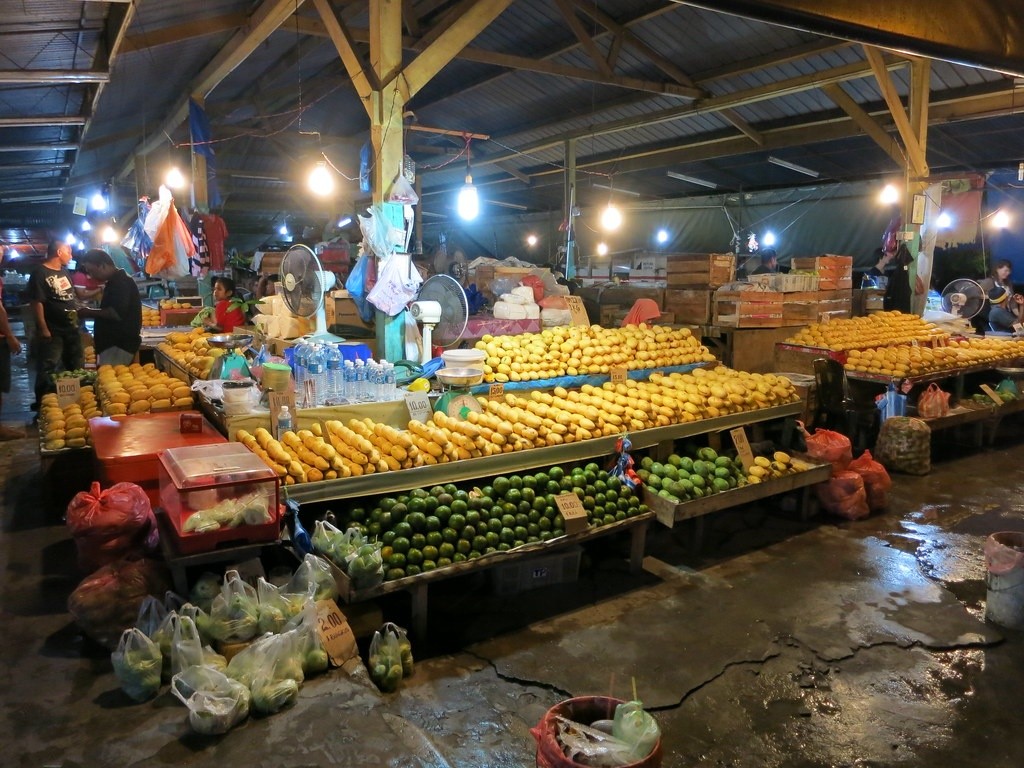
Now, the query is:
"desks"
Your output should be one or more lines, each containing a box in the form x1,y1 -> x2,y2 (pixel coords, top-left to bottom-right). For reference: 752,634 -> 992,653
88,412 -> 228,491
349,509 -> 654,629
845,356 -> 1021,397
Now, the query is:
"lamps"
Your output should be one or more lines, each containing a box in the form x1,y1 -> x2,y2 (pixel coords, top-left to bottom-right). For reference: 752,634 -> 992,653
593,183 -> 641,198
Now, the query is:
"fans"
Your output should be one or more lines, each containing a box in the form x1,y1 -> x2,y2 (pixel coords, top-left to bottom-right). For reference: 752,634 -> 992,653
941,279 -> 984,318
410,276 -> 468,368
279,244 -> 346,344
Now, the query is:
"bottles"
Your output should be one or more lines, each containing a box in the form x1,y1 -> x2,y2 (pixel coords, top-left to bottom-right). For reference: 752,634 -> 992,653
294,337 -> 396,409
277,405 -> 292,441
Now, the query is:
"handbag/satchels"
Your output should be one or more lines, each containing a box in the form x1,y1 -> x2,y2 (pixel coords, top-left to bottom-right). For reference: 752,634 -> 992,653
918,384 -> 951,417
849,449 -> 891,511
916,276 -> 924,295
64,479 -> 410,737
819,472 -> 868,521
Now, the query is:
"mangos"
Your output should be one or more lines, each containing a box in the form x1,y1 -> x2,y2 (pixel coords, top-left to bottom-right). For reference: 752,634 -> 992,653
784,311 -> 1024,377
637,447 -> 743,503
747,451 -> 806,484
971,391 -> 1016,404
39,299 -> 796,484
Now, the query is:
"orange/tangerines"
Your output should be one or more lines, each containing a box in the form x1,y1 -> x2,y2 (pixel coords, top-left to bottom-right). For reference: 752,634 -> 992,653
70,463 -> 647,734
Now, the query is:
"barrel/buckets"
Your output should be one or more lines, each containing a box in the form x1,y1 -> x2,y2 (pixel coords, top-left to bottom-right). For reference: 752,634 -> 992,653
441,349 -> 487,387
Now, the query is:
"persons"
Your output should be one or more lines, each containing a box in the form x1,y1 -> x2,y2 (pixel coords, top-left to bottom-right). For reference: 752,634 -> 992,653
870,248 -> 891,277
621,298 -> 661,329
977,260 -> 1024,333
201,273 -> 285,334
752,249 -> 790,274
0,239 -> 143,440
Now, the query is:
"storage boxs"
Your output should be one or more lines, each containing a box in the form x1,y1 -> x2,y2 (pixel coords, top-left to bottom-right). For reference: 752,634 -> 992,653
156,441 -> 279,555
575,255 -> 666,288
666,252 -> 852,329
475,265 -> 550,296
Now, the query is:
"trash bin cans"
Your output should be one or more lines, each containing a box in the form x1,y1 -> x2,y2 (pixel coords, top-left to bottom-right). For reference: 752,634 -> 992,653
531,694 -> 665,768
984,531 -> 1024,634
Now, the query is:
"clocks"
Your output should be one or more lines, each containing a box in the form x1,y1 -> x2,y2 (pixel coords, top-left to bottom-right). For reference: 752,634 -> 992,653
668,171 -> 719,191
768,156 -> 821,178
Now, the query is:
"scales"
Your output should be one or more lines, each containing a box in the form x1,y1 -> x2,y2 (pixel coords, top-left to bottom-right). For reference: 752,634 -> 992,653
206,334 -> 254,380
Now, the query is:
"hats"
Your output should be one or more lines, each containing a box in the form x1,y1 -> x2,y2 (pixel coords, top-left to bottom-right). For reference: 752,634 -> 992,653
989,287 -> 1009,303
